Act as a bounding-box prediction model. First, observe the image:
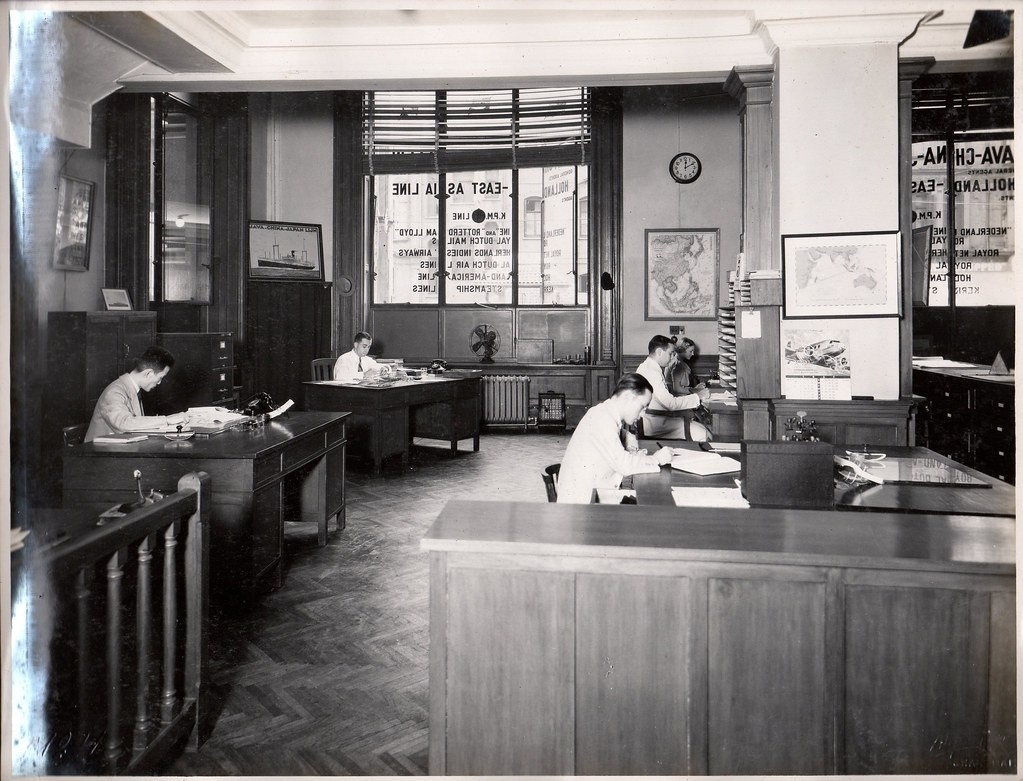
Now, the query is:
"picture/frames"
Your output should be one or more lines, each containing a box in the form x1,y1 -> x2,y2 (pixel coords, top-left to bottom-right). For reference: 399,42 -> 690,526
781,229 -> 905,320
644,228 -> 720,321
52,173 -> 96,273
246,220 -> 325,282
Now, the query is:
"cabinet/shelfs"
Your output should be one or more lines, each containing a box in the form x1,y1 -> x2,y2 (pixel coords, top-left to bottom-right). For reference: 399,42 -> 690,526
420,499 -> 1016,776
717,304 -> 781,400
39,310 -> 157,504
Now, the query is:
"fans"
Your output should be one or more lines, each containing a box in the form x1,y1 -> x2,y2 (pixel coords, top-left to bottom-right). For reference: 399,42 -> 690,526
468,323 -> 502,364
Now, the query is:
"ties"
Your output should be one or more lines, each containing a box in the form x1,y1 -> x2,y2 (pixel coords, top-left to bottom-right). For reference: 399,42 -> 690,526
358,357 -> 363,372
137,390 -> 141,406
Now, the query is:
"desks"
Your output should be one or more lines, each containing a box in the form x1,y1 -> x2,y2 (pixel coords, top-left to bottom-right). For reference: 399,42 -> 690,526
62,407 -> 353,597
912,357 -> 1014,453
302,374 -> 483,474
629,439 -> 1015,515
11,501 -> 129,583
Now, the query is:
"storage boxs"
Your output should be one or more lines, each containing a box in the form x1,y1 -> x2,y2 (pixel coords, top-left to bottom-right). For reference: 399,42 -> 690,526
156,332 -> 237,410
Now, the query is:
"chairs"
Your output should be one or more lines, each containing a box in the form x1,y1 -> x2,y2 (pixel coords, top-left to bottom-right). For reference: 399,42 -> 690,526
311,357 -> 337,381
540,463 -> 562,502
911,372 -> 979,465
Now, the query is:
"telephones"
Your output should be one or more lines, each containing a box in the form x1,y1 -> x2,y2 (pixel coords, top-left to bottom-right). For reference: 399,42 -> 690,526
427,359 -> 448,374
245,392 -> 274,416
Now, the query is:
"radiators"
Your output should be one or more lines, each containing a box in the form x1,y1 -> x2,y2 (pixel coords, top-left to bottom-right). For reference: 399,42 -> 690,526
479,375 -> 537,429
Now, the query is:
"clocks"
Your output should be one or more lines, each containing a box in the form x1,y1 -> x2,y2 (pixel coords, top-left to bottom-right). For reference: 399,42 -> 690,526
669,152 -> 702,184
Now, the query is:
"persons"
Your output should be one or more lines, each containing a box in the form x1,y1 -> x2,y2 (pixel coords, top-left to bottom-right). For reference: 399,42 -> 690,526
333,332 -> 392,380
84,346 -> 190,442
556,372 -> 678,505
664,337 -> 707,397
635,335 -> 711,442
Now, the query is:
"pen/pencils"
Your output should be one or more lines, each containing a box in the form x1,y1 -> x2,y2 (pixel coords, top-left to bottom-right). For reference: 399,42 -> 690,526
705,386 -> 707,388
657,442 -> 662,448
